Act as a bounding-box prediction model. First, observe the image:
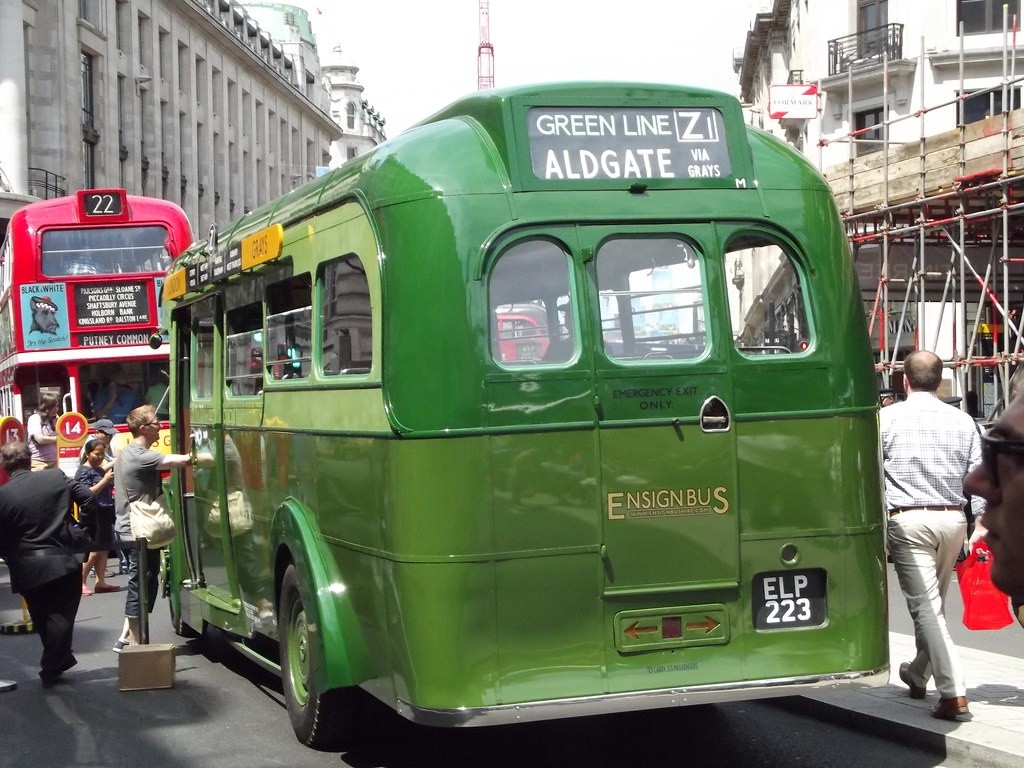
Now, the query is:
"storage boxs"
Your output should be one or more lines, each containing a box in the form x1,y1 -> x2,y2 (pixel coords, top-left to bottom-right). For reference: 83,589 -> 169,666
119,644 -> 175,691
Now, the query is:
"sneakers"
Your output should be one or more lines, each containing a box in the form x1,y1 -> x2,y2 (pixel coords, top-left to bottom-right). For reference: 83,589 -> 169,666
95,584 -> 120,592
81,588 -> 92,596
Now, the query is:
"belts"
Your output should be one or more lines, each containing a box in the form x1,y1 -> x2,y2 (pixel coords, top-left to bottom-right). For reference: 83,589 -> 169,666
889,504 -> 965,517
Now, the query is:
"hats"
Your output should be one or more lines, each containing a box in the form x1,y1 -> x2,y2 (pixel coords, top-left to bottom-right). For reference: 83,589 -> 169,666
879,388 -> 894,397
941,397 -> 962,409
94,419 -> 119,434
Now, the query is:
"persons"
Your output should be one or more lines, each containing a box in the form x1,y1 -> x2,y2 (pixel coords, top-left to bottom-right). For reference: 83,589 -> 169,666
879,351 -> 990,717
963,363 -> 1024,598
145,369 -> 169,421
74,439 -> 120,596
28,393 -> 59,471
113,406 -> 193,653
198,429 -> 263,606
78,419 -> 119,577
0,442 -> 98,688
541,301 -> 613,362
63,242 -> 104,275
94,370 -> 141,424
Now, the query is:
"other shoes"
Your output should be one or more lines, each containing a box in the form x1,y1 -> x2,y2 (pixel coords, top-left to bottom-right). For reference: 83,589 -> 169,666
61,649 -> 78,671
113,638 -> 129,652
39,667 -> 64,688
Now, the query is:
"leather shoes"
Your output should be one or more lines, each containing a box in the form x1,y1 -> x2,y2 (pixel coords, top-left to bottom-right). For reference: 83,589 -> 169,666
928,696 -> 972,721
899,662 -> 927,698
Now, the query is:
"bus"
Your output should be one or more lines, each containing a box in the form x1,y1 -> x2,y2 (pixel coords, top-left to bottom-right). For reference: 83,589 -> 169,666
495,305 -> 567,364
151,82 -> 891,749
0,187 -> 194,497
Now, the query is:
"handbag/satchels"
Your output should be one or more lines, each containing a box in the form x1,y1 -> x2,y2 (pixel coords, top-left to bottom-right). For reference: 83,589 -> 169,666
204,490 -> 253,538
956,538 -> 1014,630
129,494 -> 177,544
58,516 -> 92,547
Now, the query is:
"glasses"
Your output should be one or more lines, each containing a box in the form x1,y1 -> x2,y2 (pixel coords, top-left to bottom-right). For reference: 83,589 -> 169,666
145,420 -> 160,426
976,422 -> 1024,489
116,396 -> 124,407
